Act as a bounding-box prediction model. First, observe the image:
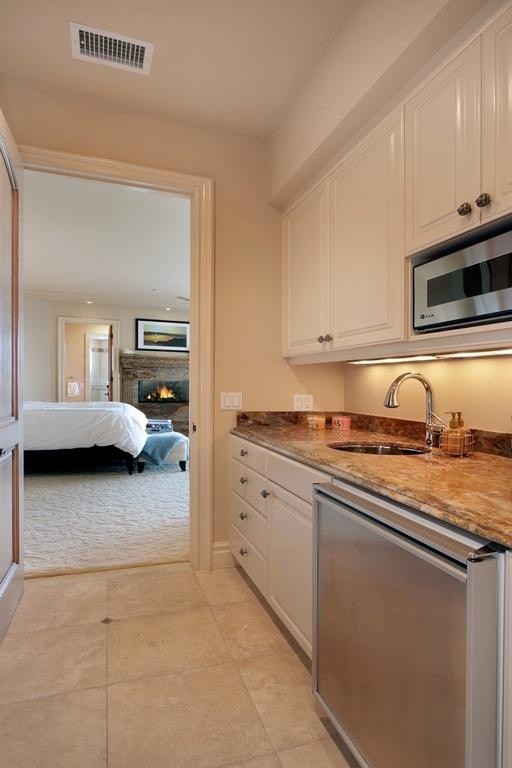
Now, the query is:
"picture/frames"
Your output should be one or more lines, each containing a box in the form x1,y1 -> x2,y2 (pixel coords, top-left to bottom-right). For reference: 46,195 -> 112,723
135,317 -> 190,353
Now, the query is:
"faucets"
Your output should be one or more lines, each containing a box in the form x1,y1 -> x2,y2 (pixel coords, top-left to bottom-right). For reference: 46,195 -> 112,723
384,368 -> 442,447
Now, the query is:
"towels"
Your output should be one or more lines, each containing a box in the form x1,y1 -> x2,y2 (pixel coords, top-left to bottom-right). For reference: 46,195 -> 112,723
67,382 -> 79,397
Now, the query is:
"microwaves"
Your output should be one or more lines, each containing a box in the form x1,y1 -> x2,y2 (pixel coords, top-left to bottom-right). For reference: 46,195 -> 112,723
410,227 -> 512,335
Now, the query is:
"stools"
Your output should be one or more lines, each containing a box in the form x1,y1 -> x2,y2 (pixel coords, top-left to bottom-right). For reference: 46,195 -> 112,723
137,431 -> 190,473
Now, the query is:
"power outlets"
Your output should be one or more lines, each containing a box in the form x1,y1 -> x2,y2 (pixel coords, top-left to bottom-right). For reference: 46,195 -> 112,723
293,393 -> 314,411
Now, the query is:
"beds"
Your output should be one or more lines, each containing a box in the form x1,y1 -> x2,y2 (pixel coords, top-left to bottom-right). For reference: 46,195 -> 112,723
23,400 -> 148,474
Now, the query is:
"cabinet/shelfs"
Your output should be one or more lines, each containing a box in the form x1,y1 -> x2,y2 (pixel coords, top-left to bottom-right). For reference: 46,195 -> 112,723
407,0 -> 512,256
267,450 -> 333,663
227,430 -> 268,660
282,102 -> 407,367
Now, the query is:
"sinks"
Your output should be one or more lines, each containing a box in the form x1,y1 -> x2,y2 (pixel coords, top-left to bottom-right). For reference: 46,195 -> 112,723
320,439 -> 429,459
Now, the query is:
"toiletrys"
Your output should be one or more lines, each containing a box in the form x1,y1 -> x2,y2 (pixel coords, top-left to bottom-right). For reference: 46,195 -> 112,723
438,408 -> 473,456
